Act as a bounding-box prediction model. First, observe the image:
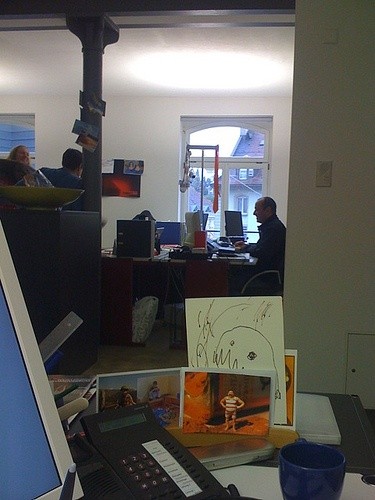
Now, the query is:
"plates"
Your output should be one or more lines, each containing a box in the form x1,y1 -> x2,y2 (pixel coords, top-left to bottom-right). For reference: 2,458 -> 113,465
0,185 -> 84,210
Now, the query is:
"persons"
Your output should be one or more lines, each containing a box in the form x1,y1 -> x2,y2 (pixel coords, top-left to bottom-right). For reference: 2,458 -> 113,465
36,148 -> 83,211
6,145 -> 30,165
117,386 -> 135,407
220,390 -> 245,432
228,197 -> 286,295
149,381 -> 160,400
125,162 -> 143,174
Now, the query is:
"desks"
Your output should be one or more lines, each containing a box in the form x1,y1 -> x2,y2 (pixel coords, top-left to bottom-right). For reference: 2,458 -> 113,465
102,249 -> 265,350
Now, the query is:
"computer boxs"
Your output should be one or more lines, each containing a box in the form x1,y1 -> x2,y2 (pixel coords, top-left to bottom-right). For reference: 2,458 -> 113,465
117,219 -> 155,258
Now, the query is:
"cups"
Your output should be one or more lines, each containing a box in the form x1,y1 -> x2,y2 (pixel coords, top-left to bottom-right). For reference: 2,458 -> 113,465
278,437 -> 346,500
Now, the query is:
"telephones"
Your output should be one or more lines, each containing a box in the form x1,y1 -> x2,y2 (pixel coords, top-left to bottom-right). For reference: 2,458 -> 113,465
207,238 -> 221,250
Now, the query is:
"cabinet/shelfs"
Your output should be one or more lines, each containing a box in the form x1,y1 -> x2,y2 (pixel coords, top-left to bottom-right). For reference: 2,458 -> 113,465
0,208 -> 102,375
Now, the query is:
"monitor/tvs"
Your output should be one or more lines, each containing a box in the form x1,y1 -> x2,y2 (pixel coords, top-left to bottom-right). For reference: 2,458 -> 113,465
155,221 -> 181,249
1,231 -> 85,500
223,211 -> 244,237
185,210 -> 209,246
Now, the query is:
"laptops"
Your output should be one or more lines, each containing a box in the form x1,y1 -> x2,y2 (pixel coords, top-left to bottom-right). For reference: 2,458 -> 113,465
206,239 -> 237,252
